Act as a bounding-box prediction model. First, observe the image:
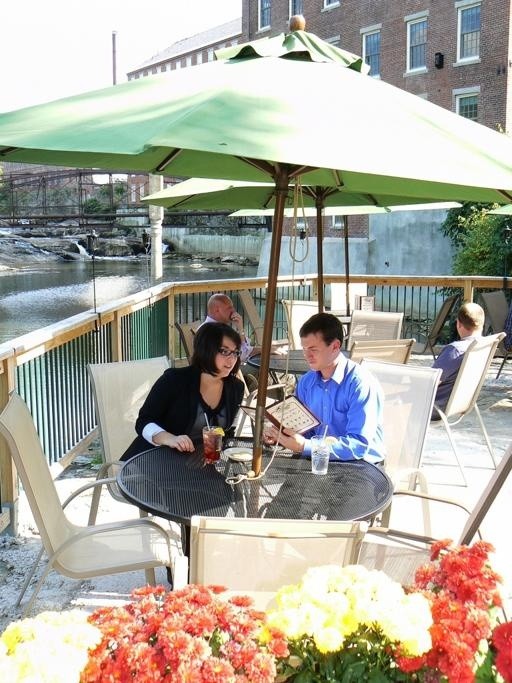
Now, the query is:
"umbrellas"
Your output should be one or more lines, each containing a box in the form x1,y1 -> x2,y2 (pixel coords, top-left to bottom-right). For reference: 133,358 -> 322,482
227,202 -> 463,324
0,13 -> 511,471
141,175 -> 483,314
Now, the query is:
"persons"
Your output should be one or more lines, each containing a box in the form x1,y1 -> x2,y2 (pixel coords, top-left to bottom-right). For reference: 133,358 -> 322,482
426,303 -> 486,420
192,292 -> 287,362
254,312 -> 389,519
121,322 -> 245,588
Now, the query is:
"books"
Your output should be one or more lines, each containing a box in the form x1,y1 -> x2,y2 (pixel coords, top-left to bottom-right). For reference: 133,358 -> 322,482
238,395 -> 322,448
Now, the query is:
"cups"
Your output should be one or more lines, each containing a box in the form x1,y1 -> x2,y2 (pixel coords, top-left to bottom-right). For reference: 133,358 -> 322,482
202,425 -> 222,464
311,436 -> 330,475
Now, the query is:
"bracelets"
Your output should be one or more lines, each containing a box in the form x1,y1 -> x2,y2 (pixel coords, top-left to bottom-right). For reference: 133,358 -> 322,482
236,330 -> 248,336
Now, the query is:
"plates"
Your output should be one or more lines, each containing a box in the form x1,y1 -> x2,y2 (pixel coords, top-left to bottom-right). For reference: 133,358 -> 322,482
223,447 -> 254,462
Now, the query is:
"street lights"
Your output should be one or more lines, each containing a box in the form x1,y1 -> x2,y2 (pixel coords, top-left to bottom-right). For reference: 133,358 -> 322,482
140,227 -> 153,293
85,227 -> 100,313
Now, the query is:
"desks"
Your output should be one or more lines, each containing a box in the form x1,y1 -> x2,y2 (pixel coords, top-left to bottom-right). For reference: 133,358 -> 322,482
116,438 -> 393,521
248,349 -> 379,450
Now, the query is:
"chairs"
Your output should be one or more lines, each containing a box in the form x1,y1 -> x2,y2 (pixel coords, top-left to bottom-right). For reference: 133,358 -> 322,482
406,294 -> 459,359
189,515 -> 368,591
435,332 -> 507,486
0,390 -> 176,619
360,359 -> 443,570
349,338 -> 416,365
282,300 -> 324,349
477,290 -> 512,380
239,289 -> 264,346
342,442 -> 512,586
175,318 -> 202,366
87,356 -> 181,583
346,310 -> 404,352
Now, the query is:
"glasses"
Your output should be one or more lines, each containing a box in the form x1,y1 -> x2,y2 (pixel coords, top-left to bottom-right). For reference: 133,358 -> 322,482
220,348 -> 243,357
